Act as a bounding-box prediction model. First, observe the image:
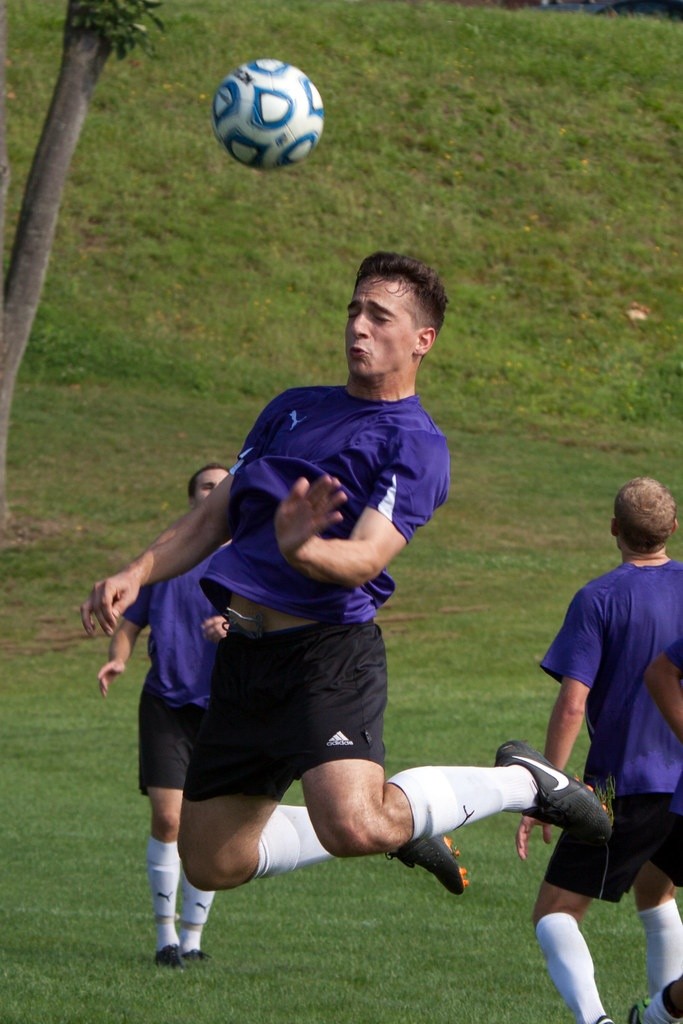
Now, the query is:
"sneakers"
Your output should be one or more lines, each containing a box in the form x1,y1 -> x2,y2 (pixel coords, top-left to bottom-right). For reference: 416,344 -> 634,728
384,834 -> 467,895
156,945 -> 184,971
182,949 -> 209,961
494,741 -> 612,848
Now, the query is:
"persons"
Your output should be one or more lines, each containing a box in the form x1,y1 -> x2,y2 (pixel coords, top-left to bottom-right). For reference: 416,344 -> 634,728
96,464 -> 230,969
514,477 -> 683,1024
79,249 -> 614,896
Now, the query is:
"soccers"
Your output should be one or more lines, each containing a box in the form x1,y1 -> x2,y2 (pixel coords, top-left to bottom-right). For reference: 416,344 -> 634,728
211,57 -> 324,171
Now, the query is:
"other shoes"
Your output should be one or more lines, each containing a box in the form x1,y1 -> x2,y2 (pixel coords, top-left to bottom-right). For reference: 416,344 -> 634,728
628,999 -> 654,1024
